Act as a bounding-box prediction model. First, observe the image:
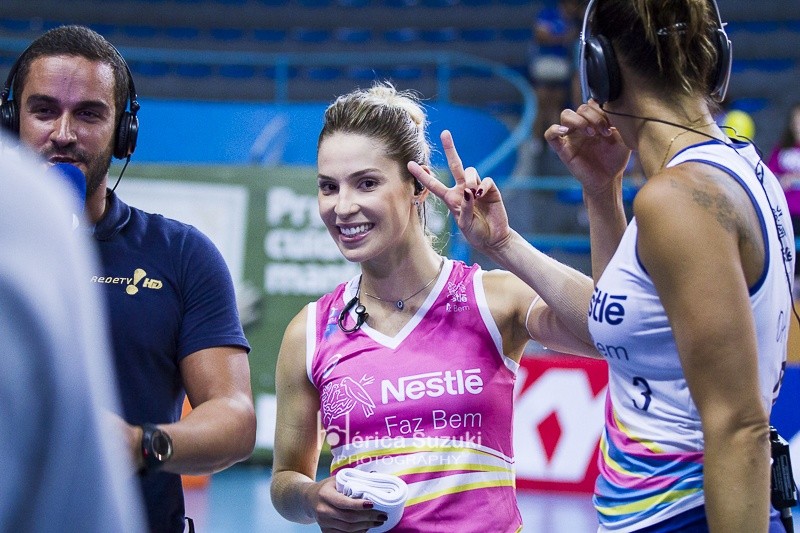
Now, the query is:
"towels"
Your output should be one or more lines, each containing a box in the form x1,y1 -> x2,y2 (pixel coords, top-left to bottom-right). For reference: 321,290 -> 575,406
335,467 -> 409,533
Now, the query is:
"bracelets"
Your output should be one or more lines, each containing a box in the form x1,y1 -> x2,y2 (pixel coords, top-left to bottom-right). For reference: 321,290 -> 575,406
525,296 -> 540,341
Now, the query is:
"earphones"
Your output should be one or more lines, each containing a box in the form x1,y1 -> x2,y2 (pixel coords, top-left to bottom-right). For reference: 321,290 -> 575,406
355,305 -> 366,331
414,177 -> 424,196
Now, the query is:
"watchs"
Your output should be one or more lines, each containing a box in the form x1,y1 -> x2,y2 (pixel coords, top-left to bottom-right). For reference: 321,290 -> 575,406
140,424 -> 174,469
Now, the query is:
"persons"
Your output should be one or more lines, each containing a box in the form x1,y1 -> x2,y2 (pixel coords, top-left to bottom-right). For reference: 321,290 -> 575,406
545,1 -> 800,533
268,82 -> 605,533
0,132 -> 149,533
0,25 -> 257,533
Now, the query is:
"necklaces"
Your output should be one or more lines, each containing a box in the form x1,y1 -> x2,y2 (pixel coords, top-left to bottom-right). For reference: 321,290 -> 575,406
360,260 -> 444,309
658,122 -> 717,171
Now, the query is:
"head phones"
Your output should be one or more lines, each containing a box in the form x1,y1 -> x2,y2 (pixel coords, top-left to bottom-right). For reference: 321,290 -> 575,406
579,0 -> 734,104
0,39 -> 140,160
